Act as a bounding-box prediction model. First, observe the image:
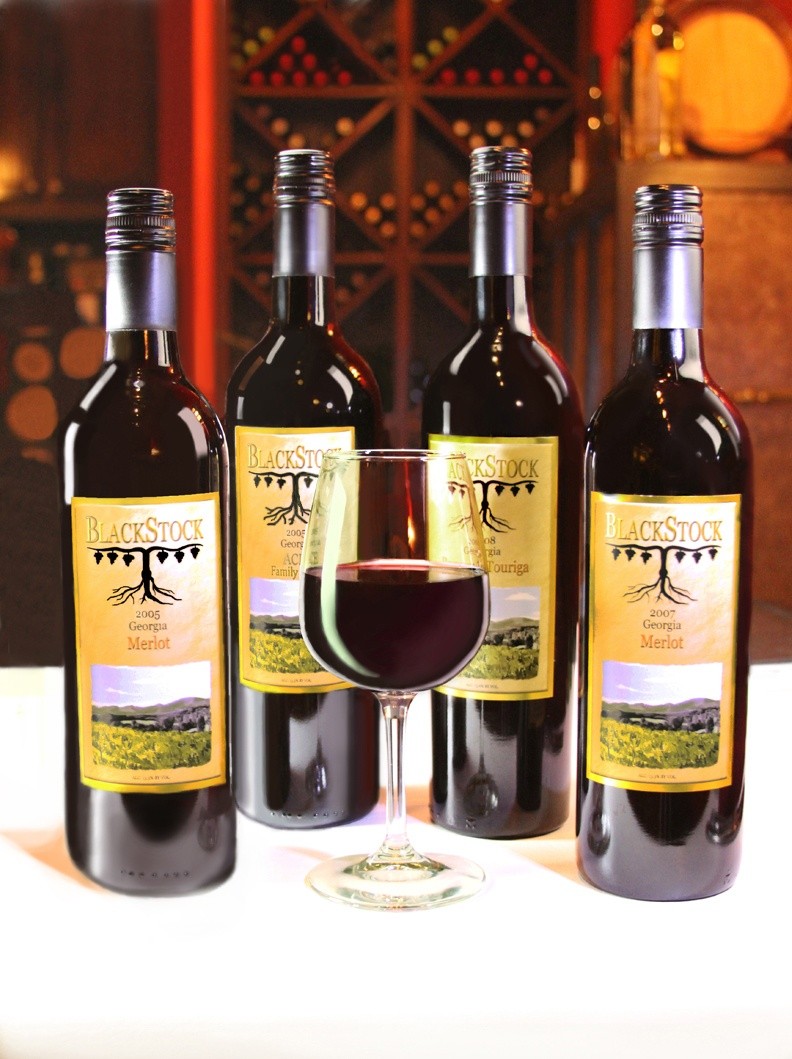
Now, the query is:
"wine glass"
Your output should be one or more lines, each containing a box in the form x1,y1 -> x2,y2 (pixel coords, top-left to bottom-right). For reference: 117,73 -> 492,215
295,446 -> 488,911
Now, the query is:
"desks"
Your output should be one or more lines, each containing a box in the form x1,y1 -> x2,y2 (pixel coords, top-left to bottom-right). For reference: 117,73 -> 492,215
0,653 -> 792,1053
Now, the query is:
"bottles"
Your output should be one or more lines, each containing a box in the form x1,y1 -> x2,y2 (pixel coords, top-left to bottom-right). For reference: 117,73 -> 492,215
417,144 -> 583,842
581,178 -> 750,900
225,144 -> 378,831
60,185 -> 237,894
